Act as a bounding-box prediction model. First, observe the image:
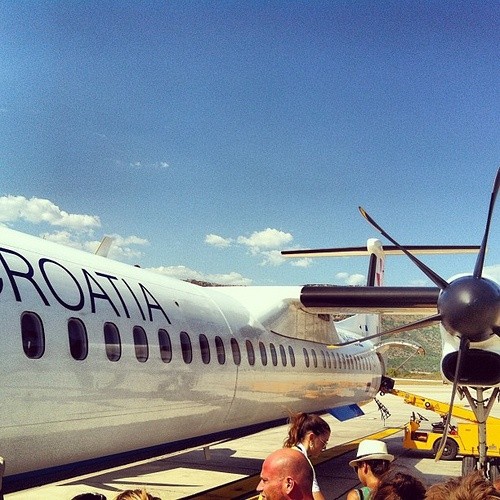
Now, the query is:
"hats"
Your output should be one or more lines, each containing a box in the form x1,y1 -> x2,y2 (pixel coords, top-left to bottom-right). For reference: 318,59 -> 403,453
350,440 -> 395,465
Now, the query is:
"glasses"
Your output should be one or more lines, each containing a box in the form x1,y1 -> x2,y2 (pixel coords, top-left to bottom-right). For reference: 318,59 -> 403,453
315,436 -> 329,449
353,466 -> 358,473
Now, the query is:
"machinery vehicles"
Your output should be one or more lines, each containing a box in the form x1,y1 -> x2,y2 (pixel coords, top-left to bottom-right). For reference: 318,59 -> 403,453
376,376 -> 500,460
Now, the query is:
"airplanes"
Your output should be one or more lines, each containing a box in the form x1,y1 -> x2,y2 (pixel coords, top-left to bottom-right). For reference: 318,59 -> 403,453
0,165 -> 500,477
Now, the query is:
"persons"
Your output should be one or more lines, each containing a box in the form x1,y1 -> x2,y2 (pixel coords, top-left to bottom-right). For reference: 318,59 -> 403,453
283,412 -> 331,500
256,448 -> 314,500
347,439 -> 500,500
112,489 -> 162,500
